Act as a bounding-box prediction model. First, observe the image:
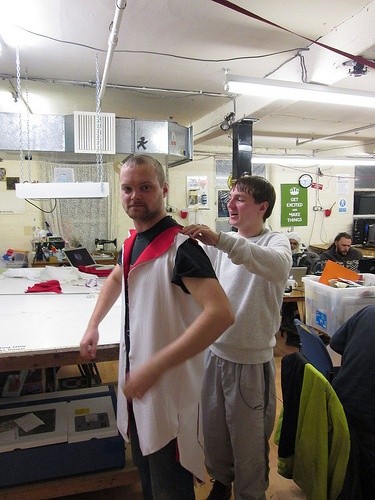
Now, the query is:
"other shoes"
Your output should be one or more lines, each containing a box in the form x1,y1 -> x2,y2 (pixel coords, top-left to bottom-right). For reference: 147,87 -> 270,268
205,479 -> 232,500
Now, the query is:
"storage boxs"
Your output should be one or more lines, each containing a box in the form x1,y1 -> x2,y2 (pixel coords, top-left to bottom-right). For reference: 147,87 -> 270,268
301,273 -> 375,337
0,384 -> 126,486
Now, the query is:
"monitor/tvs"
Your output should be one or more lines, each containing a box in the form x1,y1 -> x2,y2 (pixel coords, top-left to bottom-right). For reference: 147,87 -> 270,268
366,224 -> 375,248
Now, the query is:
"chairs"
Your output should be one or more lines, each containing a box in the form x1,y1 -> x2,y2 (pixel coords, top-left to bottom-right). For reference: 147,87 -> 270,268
272,319 -> 351,500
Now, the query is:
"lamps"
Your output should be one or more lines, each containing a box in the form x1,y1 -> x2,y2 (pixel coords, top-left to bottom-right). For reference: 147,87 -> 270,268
14,47 -> 109,199
220,112 -> 236,131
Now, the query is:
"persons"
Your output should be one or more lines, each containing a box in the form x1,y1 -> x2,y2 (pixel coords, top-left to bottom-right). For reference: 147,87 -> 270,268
180,176 -> 292,500
311,233 -> 363,276
289,233 -> 311,267
80,155 -> 235,500
329,304 -> 375,500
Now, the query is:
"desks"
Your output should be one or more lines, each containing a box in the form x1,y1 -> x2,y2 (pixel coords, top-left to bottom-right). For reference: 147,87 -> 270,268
30,252 -> 116,268
283,290 -> 306,323
0,268 -> 142,500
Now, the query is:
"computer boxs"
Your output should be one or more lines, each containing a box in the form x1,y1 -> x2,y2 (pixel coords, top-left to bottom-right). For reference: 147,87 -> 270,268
352,218 -> 375,245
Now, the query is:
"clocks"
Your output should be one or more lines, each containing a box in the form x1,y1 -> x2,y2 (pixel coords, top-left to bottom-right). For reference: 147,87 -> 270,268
299,174 -> 313,188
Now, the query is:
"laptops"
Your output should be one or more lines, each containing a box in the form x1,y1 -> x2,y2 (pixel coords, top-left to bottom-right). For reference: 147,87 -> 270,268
287,267 -> 307,287
64,247 -> 114,271
